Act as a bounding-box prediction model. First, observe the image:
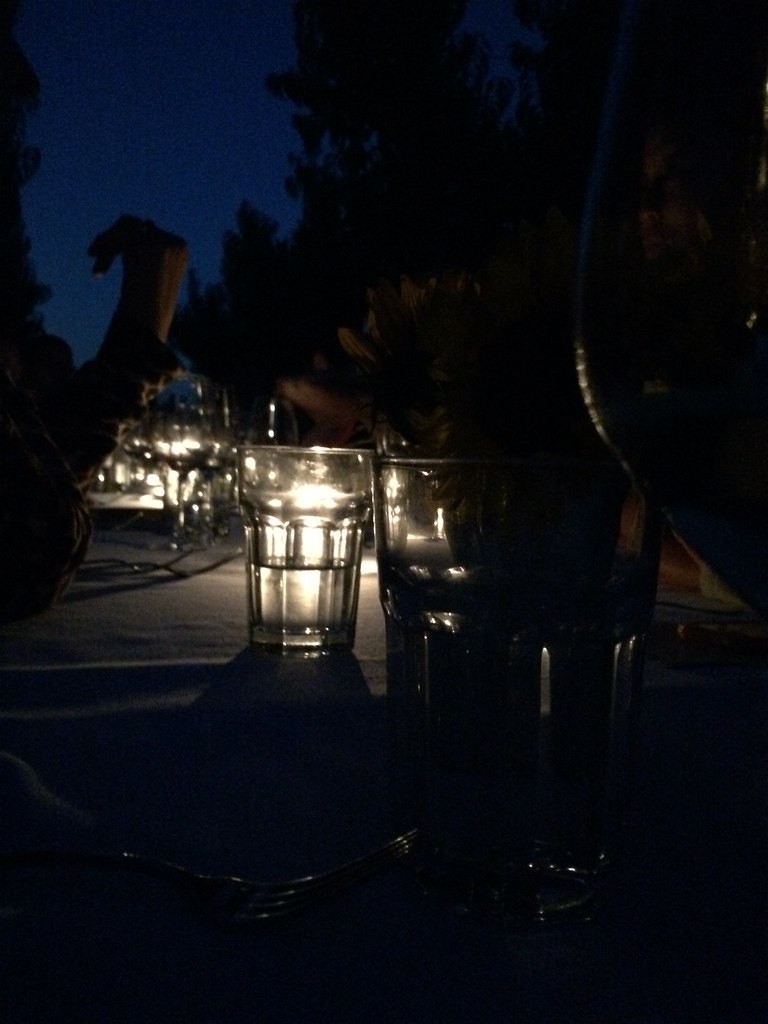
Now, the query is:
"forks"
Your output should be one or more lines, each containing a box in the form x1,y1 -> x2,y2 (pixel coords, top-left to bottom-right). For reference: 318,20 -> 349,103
0,822 -> 419,931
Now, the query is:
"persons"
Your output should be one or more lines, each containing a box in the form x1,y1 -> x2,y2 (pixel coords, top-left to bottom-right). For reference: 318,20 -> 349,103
282,115 -> 713,593
156,360 -> 201,405
0,213 -> 189,623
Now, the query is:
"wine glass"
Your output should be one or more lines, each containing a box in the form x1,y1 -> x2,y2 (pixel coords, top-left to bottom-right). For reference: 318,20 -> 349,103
150,385 -> 225,552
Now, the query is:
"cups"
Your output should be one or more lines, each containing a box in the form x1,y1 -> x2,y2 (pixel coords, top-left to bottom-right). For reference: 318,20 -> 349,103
240,396 -> 299,524
370,458 -> 474,635
381,456 -> 643,930
238,444 -> 374,648
